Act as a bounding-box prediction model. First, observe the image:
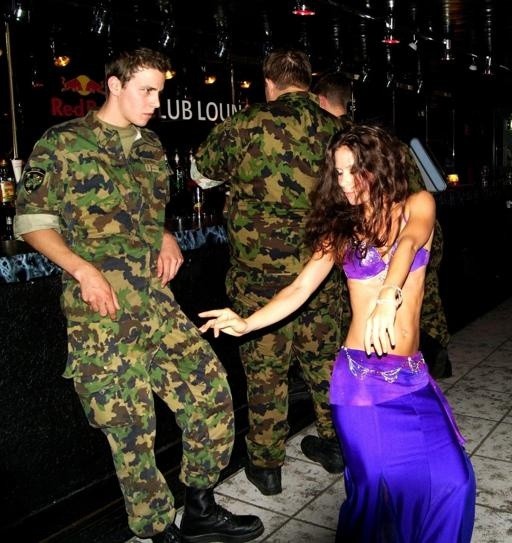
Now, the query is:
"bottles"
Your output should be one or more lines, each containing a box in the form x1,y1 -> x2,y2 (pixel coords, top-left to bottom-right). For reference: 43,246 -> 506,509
170,146 -> 206,213
0,157 -> 18,258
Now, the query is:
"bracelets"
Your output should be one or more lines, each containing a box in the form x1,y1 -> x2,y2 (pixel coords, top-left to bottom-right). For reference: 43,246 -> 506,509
374,284 -> 404,306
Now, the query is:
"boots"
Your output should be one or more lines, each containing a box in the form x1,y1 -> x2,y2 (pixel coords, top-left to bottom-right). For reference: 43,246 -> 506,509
151,486 -> 264,543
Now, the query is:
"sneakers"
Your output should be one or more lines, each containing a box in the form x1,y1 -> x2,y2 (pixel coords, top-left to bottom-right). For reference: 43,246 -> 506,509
300,434 -> 344,474
244,461 -> 282,495
417,346 -> 452,379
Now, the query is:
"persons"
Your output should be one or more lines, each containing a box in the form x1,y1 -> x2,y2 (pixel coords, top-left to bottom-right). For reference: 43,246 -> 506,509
312,74 -> 354,131
419,219 -> 453,379
197,128 -> 477,543
11,44 -> 264,543
188,47 -> 344,496
